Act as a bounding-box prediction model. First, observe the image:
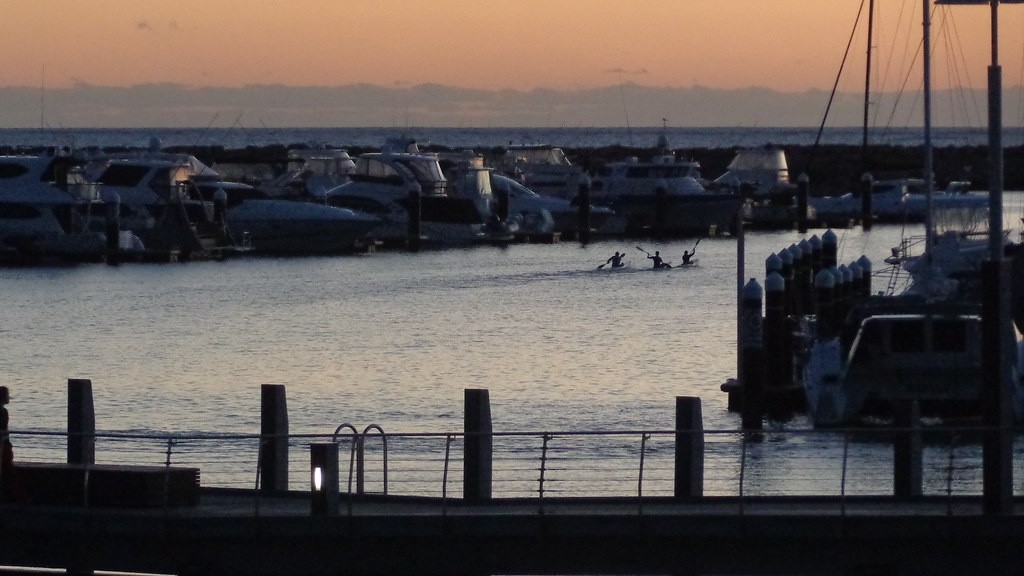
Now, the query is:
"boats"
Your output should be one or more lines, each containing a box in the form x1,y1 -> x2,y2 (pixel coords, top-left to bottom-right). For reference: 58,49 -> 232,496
0,61 -> 989,268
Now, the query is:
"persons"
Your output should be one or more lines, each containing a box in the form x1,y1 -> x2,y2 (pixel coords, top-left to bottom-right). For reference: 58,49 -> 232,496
0,386 -> 14,466
607,252 -> 625,268
682,248 -> 695,264
647,251 -> 671,268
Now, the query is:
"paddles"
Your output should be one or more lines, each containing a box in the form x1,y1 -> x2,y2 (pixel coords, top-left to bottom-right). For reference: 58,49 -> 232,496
689,236 -> 702,254
599,253 -> 625,269
636,245 -> 671,268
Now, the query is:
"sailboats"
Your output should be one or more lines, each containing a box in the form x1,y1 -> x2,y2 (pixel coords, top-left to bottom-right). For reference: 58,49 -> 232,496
811,0 -> 1024,440
